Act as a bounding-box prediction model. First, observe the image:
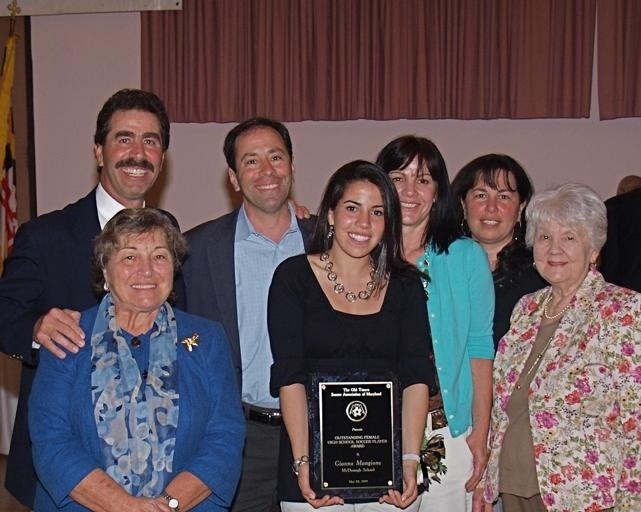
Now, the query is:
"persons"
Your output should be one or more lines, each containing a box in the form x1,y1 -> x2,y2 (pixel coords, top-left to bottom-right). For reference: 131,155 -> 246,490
1,88 -> 312,511
471,180 -> 641,512
597,185 -> 641,293
26,206 -> 249,512
616,174 -> 641,195
181,114 -> 319,512
450,151 -> 555,354
267,158 -> 439,511
375,134 -> 497,512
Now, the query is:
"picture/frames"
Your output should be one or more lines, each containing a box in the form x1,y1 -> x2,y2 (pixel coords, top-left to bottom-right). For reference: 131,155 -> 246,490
311,371 -> 403,501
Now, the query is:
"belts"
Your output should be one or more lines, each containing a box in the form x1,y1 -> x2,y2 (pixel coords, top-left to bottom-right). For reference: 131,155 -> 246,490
242,402 -> 284,427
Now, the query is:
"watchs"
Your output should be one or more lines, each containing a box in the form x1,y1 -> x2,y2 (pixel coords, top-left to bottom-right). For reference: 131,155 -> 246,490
161,490 -> 182,512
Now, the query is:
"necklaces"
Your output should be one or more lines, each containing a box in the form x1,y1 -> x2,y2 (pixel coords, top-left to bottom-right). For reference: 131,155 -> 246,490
516,321 -> 558,392
544,294 -> 574,319
319,248 -> 378,302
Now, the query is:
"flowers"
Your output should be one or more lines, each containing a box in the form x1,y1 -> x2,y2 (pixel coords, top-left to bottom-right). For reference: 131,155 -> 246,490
420,429 -> 447,490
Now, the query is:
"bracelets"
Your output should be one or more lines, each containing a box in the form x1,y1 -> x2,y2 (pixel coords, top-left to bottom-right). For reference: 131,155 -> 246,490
294,453 -> 310,473
401,452 -> 421,463
